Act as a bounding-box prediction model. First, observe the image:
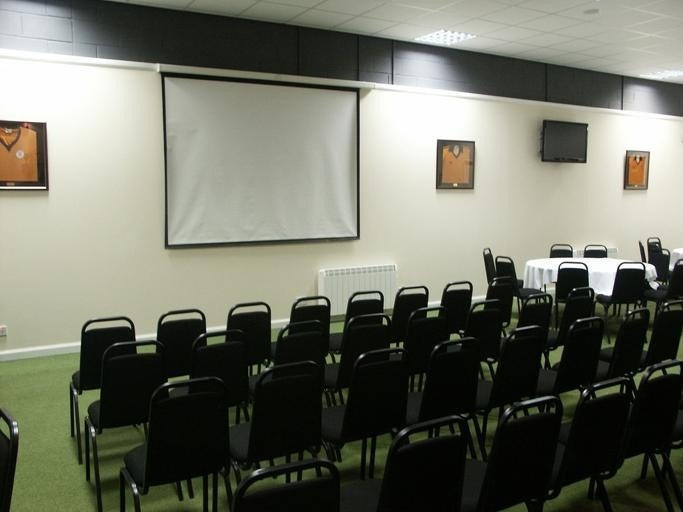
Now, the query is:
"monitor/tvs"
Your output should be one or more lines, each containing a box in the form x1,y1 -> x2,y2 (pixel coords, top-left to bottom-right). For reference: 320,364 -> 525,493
543,120 -> 588,163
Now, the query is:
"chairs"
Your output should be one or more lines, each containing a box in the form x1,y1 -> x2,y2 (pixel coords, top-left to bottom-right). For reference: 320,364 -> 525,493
0,409 -> 19,512
69,237 -> 683,512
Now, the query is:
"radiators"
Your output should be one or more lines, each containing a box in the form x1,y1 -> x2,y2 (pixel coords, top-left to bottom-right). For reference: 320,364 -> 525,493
314,262 -> 403,318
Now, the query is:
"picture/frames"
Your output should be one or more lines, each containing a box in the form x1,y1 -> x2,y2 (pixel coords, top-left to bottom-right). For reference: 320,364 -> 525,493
0,120 -> 49,191
436,140 -> 475,189
624,150 -> 650,190
